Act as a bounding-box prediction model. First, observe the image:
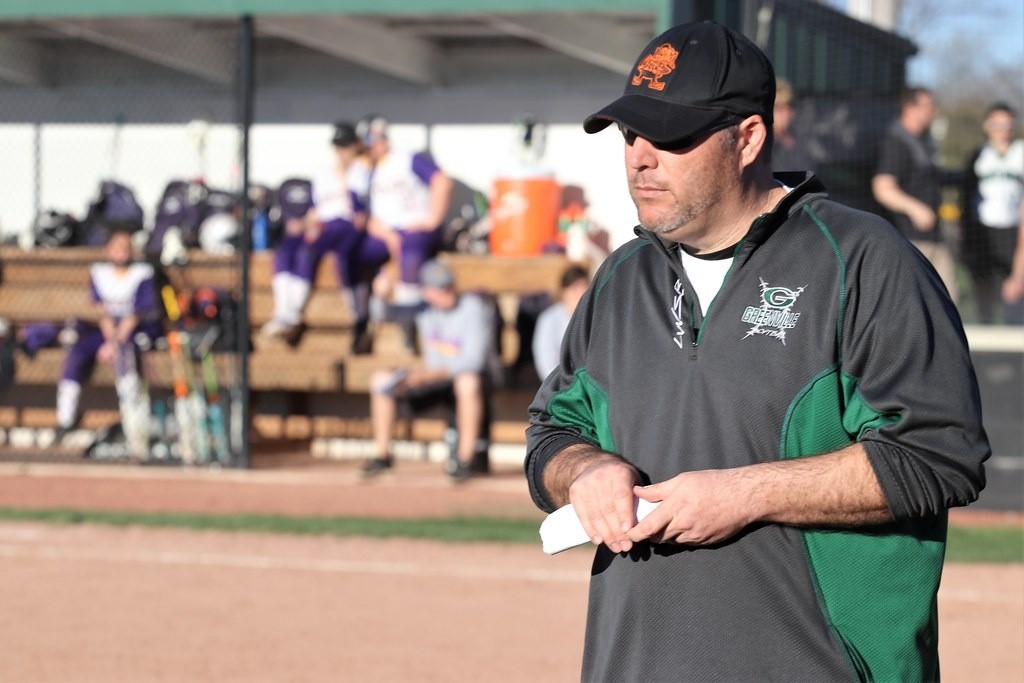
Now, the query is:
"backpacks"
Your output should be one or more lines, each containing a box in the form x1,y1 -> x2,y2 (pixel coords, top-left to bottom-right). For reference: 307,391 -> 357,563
144,181 -> 209,255
83,181 -> 144,248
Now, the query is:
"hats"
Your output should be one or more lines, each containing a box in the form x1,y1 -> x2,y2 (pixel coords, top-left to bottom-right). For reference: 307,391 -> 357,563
583,21 -> 776,144
420,261 -> 454,287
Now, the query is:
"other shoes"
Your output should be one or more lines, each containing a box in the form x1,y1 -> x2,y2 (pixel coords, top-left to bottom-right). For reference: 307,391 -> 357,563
449,458 -> 471,478
362,456 -> 391,474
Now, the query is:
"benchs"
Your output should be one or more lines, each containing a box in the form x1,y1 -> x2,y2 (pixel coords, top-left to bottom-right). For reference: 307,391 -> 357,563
0,242 -> 590,442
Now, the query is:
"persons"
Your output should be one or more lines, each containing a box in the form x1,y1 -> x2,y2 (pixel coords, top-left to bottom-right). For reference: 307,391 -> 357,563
955,101 -> 1024,324
768,76 -> 816,172
358,261 -> 498,475
259,115 -> 453,355
523,19 -> 993,682
869,88 -> 957,304
532,266 -> 590,381
50,230 -> 164,463
